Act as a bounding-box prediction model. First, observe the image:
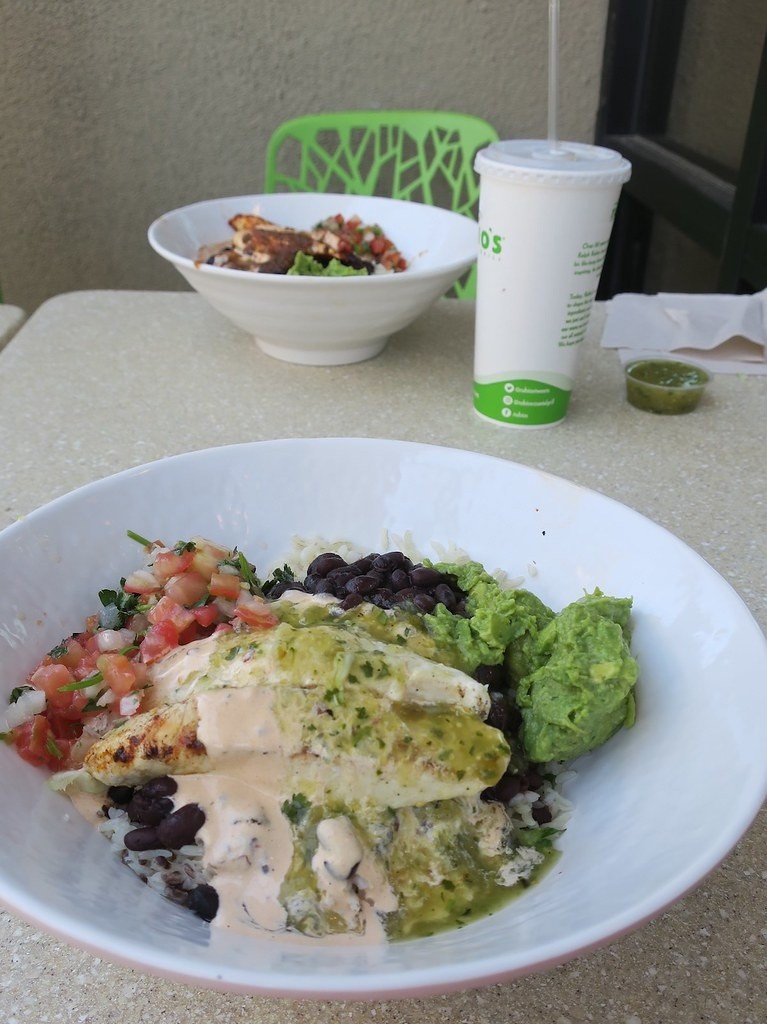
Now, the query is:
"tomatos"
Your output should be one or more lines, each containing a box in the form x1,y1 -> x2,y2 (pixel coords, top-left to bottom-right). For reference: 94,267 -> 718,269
1,541 -> 280,768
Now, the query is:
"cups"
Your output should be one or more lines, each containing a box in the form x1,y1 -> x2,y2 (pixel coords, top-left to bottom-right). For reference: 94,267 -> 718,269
472,138 -> 631,430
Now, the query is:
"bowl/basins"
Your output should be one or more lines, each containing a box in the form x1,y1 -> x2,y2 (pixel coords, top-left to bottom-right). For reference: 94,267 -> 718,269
145,190 -> 479,366
623,352 -> 714,415
0,437 -> 767,1000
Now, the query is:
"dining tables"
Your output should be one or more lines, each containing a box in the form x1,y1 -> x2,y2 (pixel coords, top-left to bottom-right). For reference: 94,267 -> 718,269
0,289 -> 767,1024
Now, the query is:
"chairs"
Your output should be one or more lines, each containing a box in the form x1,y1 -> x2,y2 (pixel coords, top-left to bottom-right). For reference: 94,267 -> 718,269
264,107 -> 499,297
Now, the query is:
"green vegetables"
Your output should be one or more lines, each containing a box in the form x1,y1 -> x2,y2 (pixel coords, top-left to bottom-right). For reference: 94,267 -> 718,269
310,218 -> 383,255
9,530 -> 295,760
